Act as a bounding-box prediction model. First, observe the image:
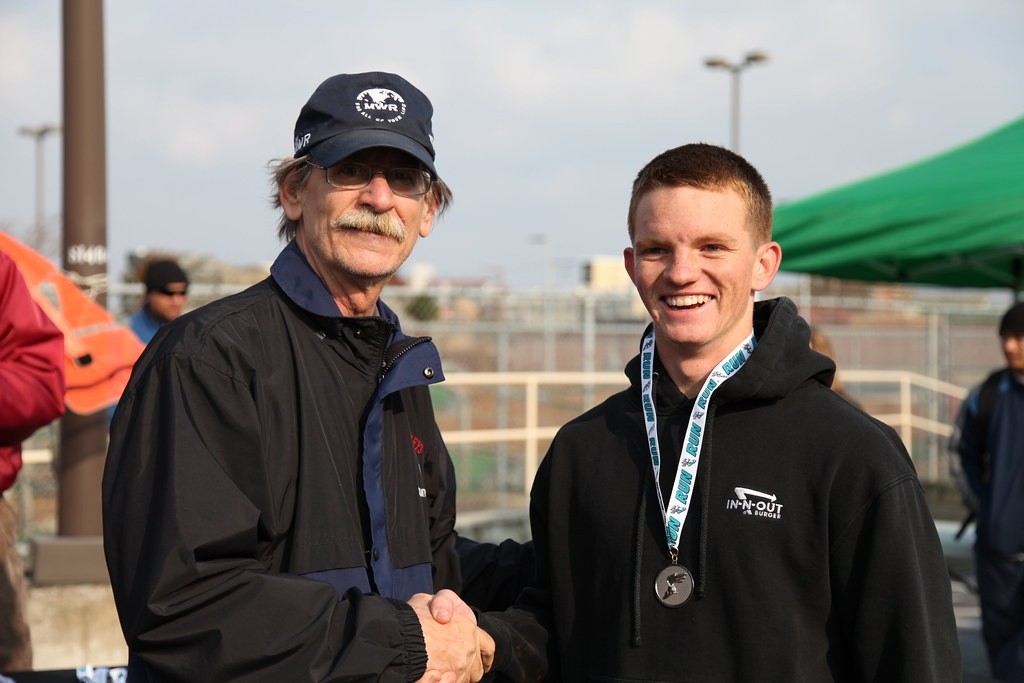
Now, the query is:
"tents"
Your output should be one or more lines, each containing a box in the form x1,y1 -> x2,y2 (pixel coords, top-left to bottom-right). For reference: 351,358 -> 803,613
754,113 -> 1024,306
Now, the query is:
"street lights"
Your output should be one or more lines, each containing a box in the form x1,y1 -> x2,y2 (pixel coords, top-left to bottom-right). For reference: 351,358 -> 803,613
16,125 -> 64,254
705,50 -> 769,152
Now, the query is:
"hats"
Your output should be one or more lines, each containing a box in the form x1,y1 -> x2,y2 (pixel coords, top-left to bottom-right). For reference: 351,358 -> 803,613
293,72 -> 437,181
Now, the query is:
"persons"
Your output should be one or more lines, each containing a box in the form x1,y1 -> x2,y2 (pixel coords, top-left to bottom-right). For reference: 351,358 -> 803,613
96,70 -> 540,683
947,302 -> 1024,683
0,248 -> 68,672
103,260 -> 195,457
407,144 -> 966,683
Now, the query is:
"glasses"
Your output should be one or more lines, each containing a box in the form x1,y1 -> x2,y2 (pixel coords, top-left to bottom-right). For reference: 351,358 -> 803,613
303,159 -> 434,198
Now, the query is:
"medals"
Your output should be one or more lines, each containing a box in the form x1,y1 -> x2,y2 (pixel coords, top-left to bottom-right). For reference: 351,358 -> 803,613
653,564 -> 695,609
